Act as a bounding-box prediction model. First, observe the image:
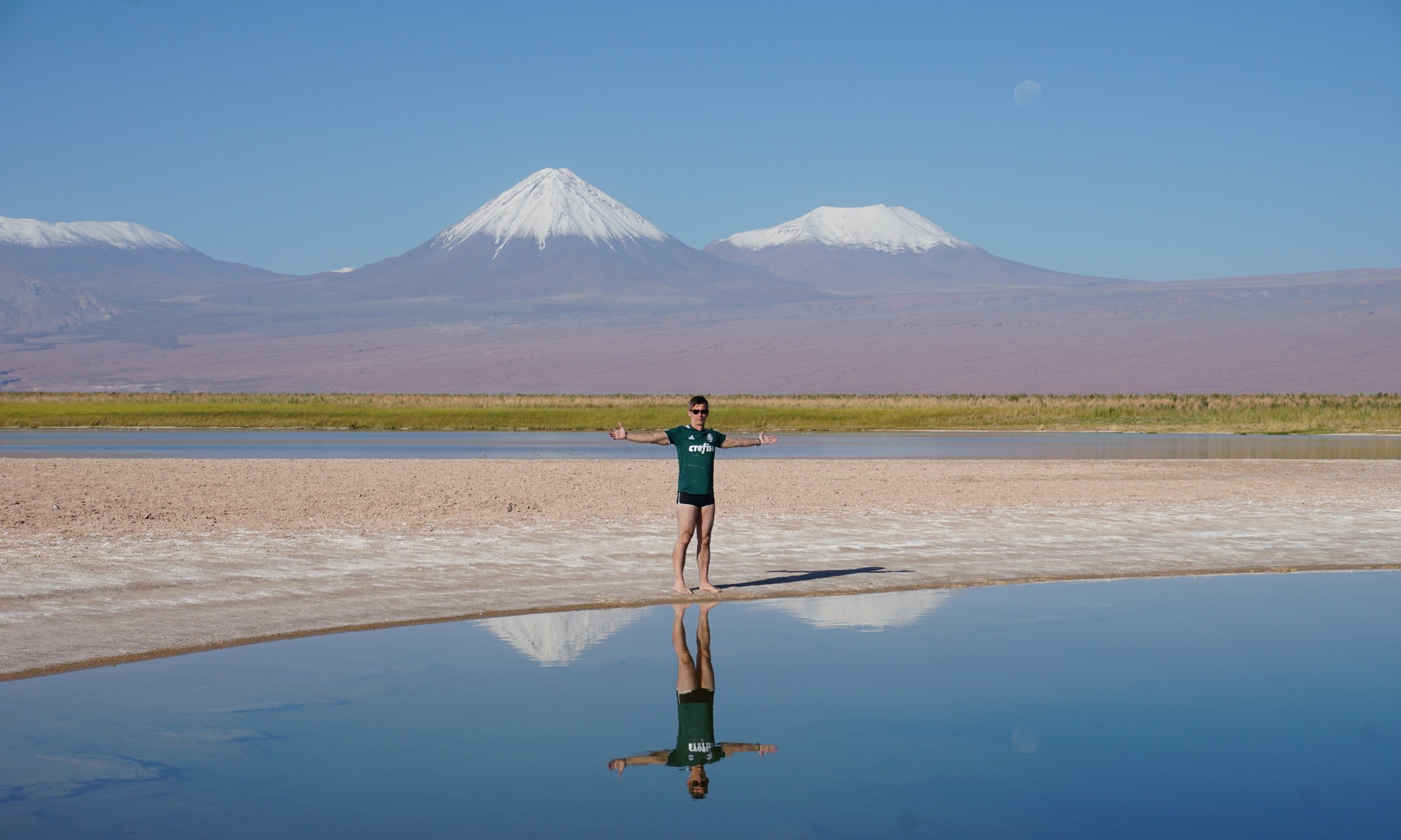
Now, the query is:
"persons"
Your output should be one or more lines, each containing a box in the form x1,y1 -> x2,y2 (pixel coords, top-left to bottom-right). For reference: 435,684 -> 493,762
608,397 -> 778,594
607,602 -> 778,799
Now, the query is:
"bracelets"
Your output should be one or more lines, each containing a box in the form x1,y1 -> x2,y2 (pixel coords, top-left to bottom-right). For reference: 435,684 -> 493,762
758,745 -> 760,751
757,438 -> 761,446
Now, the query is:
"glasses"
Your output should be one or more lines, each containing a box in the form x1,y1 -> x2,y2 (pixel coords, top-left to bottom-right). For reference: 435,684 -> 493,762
690,409 -> 708,415
691,780 -> 707,787
